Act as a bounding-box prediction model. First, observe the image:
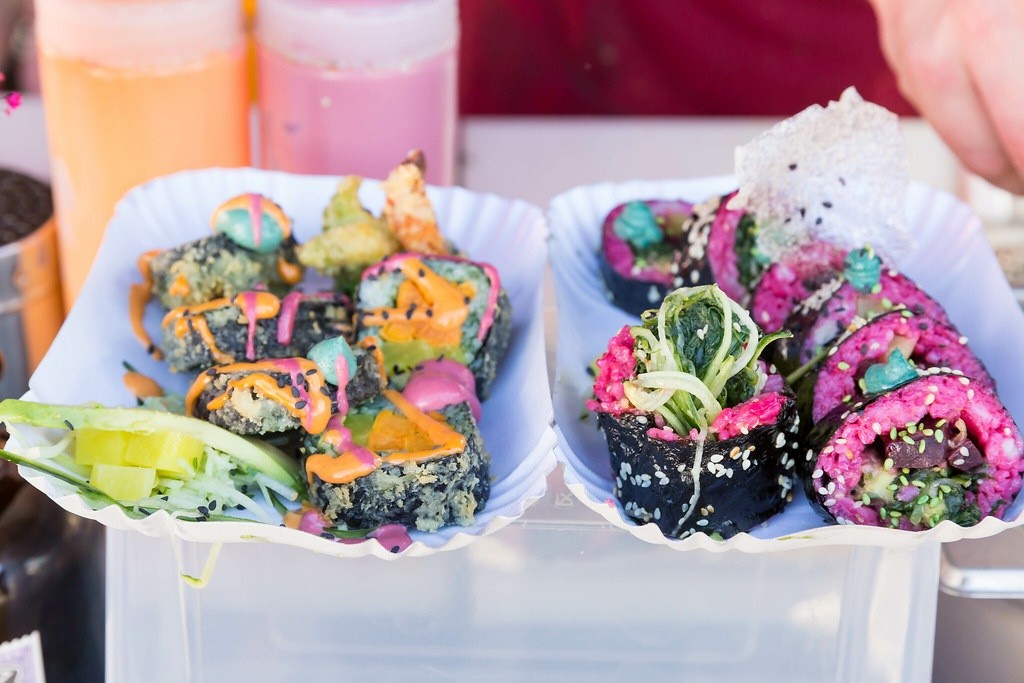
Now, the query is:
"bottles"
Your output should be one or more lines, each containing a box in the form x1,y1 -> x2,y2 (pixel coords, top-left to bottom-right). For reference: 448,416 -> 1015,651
254,1 -> 459,188
33,0 -> 251,321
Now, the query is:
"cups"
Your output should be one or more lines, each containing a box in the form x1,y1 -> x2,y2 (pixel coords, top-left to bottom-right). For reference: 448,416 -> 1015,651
0,167 -> 65,400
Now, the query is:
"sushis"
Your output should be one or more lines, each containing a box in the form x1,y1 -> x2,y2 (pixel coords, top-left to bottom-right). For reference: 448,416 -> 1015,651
151,223 -> 515,530
584,188 -> 1020,540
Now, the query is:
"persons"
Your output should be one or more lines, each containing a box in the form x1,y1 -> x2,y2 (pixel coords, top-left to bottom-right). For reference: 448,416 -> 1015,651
0,0 -> 1024,196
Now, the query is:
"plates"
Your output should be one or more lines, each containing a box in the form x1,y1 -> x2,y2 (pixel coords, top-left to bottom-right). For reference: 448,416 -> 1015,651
549,172 -> 1024,553
3,166 -> 556,559
